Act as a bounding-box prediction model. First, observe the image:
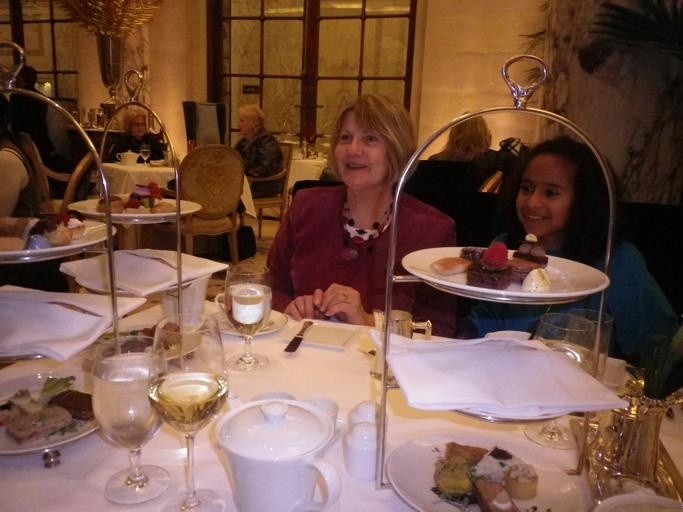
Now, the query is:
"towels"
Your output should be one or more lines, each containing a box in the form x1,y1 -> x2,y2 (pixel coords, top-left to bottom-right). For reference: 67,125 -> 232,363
0,285 -> 147,360
59,248 -> 228,298
372,330 -> 629,419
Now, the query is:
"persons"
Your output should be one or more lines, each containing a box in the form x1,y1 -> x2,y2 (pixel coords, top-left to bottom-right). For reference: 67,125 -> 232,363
0,93 -> 52,220
261,94 -> 460,338
428,114 -> 499,162
457,137 -> 682,372
0,66 -> 57,170
233,103 -> 285,227
106,105 -> 166,164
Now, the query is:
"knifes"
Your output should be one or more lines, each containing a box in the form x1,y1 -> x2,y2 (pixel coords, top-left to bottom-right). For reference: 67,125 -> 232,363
283,320 -> 315,354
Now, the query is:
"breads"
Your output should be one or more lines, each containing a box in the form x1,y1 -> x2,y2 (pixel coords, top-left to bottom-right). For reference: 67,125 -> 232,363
432,256 -> 467,275
1,216 -> 39,240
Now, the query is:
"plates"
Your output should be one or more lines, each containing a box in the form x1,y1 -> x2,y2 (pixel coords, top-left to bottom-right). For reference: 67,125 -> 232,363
440,336 -> 570,424
400,245 -> 613,306
383,431 -> 594,512
0,218 -> 118,266
74,275 -> 213,296
209,309 -> 287,338
0,360 -> 99,456
63,193 -> 202,224
79,325 -> 204,363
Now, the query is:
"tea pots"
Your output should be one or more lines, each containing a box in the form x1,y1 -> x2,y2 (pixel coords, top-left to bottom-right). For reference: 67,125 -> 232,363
116,150 -> 141,165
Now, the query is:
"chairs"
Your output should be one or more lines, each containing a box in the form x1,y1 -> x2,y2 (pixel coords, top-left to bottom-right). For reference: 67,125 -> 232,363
158,145 -> 244,264
239,143 -> 294,239
412,160 -> 480,194
292,181 -> 683,322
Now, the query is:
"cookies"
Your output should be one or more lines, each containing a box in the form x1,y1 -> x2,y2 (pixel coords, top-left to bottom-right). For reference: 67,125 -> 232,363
6,407 -> 74,447
48,388 -> 101,423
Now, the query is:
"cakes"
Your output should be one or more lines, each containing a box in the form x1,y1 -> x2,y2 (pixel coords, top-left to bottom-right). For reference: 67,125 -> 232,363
476,444 -> 524,483
474,477 -> 519,512
95,194 -> 125,215
512,244 -> 549,283
435,462 -> 470,501
159,323 -> 181,353
520,266 -> 550,293
67,217 -> 86,240
461,247 -> 484,260
466,260 -> 512,290
31,218 -> 69,245
506,465 -> 539,500
134,183 -> 157,200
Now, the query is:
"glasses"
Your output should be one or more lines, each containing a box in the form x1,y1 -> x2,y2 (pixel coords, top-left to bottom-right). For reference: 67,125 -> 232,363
131,122 -> 146,127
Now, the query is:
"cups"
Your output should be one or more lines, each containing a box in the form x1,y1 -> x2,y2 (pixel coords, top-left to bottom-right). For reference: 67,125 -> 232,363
223,264 -> 269,373
523,311 -> 595,449
569,309 -> 614,380
213,397 -> 342,512
348,399 -> 380,422
140,144 -> 151,166
373,308 -> 433,339
88,334 -> 172,506
147,316 -> 230,511
343,422 -> 379,480
156,274 -> 211,333
214,285 -> 272,324
